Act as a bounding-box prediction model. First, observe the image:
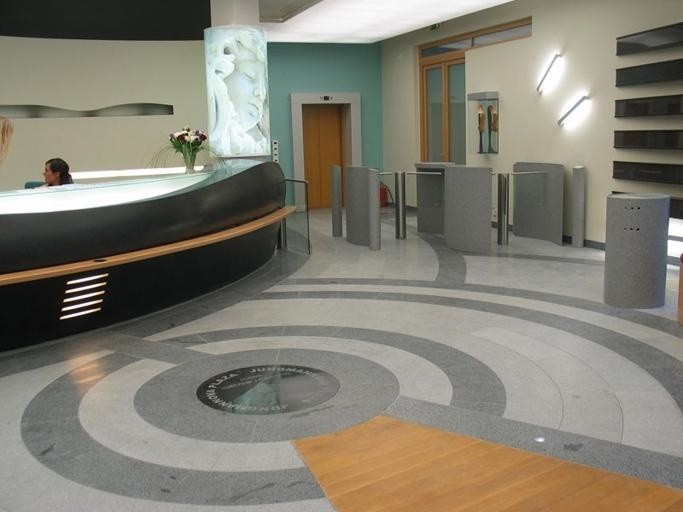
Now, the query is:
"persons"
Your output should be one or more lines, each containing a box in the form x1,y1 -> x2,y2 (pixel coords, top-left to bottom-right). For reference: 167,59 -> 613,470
39,158 -> 72,188
0,116 -> 14,165
212,28 -> 271,153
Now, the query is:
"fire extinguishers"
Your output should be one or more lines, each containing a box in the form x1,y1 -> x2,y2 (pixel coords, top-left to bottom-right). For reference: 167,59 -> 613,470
379,182 -> 394,207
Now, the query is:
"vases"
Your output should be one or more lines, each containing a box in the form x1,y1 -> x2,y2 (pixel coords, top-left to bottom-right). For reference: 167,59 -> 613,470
144,126 -> 235,174
182,151 -> 197,175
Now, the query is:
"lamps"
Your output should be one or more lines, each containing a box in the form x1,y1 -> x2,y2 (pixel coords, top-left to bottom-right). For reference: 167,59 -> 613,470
536,53 -> 562,94
558,95 -> 592,129
